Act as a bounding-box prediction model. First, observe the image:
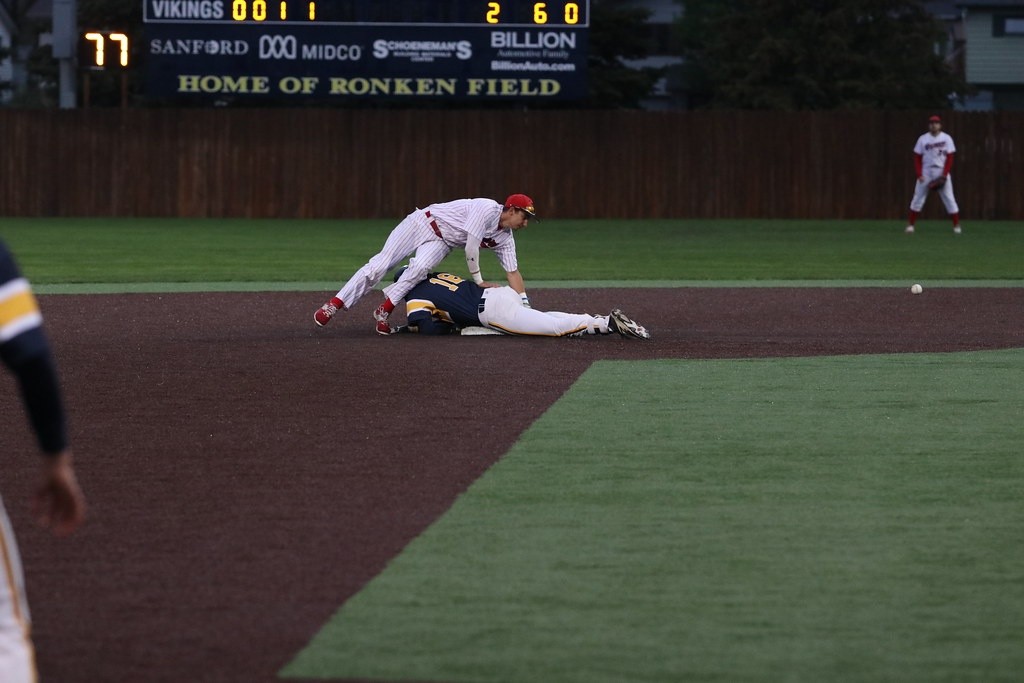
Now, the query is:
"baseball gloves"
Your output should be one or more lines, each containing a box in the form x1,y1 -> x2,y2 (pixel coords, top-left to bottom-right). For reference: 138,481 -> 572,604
928,176 -> 946,191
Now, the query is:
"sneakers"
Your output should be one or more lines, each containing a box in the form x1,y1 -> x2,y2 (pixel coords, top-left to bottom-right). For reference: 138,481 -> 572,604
374,304 -> 391,335
607,308 -> 652,340
594,314 -> 608,319
313,297 -> 344,326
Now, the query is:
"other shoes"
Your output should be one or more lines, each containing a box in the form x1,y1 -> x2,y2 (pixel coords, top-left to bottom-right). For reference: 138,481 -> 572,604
954,226 -> 961,233
906,225 -> 914,233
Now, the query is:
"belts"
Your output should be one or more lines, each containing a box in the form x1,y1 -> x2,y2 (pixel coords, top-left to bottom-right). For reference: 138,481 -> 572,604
478,298 -> 485,313
426,211 -> 443,238
930,165 -> 939,168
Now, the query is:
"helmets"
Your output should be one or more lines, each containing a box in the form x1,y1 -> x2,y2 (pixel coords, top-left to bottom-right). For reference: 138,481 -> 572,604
394,265 -> 409,282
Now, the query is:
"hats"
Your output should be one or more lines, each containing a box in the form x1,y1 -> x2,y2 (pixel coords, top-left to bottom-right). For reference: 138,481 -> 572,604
928,115 -> 941,121
505,194 -> 541,224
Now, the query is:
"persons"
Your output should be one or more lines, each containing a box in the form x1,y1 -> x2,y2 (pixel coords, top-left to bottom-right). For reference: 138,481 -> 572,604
0,237 -> 94,683
393,265 -> 652,340
313,194 -> 541,336
905,116 -> 962,234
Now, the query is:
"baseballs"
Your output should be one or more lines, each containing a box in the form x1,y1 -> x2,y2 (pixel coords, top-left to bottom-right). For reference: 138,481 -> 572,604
911,283 -> 922,295
